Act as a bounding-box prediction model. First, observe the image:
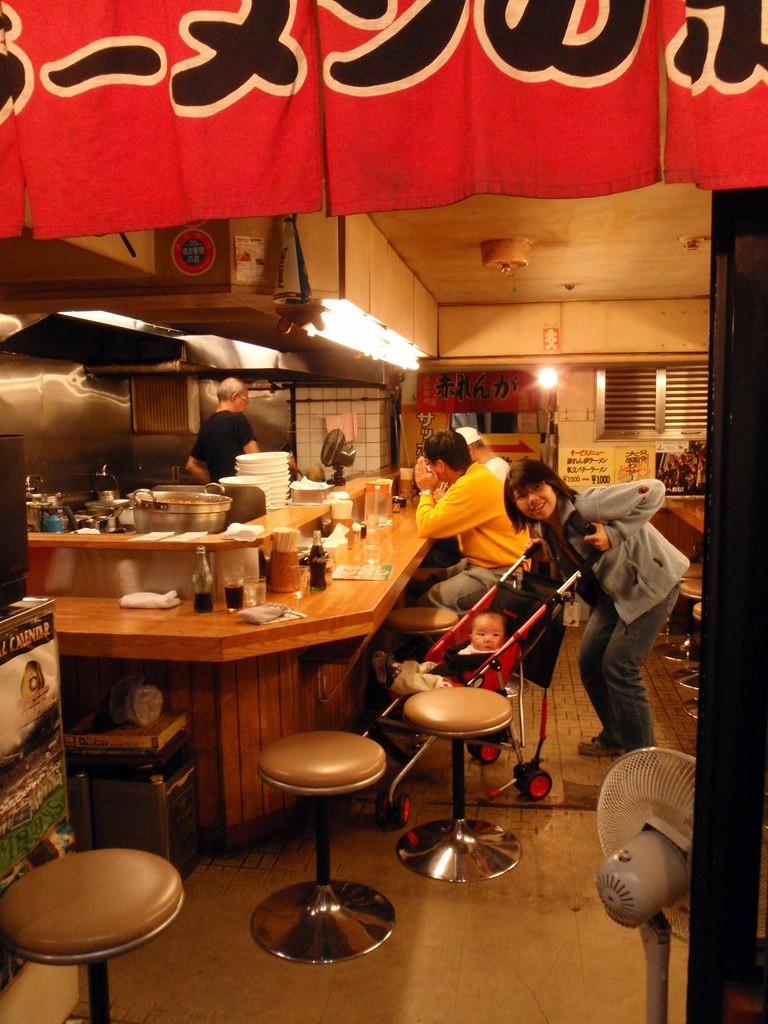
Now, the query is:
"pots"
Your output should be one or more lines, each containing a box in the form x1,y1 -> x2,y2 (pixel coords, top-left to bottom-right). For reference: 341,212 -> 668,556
128,483 -> 233,534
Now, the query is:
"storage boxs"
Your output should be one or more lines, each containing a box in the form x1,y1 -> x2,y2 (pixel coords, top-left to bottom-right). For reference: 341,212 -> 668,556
65,713 -> 190,757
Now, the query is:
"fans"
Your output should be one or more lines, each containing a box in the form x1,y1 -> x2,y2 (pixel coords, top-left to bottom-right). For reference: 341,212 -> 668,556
321,427 -> 355,486
596,745 -> 697,1024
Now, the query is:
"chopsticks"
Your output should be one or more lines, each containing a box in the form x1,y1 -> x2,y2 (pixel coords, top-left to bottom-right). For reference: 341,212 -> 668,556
377,479 -> 394,491
272,527 -> 300,552
399,468 -> 414,479
332,501 -> 353,519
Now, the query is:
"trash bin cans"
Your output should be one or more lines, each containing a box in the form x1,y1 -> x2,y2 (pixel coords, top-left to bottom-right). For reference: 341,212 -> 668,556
64,713 -> 198,884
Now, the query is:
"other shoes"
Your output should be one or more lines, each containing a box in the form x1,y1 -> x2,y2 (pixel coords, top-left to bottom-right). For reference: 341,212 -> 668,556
578,737 -> 623,756
372,651 -> 402,688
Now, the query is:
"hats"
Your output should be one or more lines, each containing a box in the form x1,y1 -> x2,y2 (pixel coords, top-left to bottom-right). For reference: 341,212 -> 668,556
456,427 -> 480,445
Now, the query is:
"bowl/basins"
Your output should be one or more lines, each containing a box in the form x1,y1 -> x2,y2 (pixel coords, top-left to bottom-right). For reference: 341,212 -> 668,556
219,450 -> 289,516
84,498 -> 131,517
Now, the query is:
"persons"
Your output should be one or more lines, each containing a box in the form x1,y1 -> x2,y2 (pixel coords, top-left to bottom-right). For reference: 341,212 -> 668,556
405,426 -> 531,616
373,607 -> 503,696
503,459 -> 689,772
186,378 -> 296,487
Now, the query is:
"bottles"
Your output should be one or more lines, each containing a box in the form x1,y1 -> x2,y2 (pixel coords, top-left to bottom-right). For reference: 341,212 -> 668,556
193,545 -> 214,613
393,498 -> 400,513
309,530 -> 326,591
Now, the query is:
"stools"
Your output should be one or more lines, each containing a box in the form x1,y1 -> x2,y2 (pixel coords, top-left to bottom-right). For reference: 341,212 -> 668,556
248,729 -> 396,964
395,687 -> 522,883
381,606 -> 460,749
656,561 -> 705,718
0,846 -> 185,1024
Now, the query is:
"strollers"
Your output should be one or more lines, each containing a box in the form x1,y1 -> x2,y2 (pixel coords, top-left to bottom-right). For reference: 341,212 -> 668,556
360,519 -> 603,823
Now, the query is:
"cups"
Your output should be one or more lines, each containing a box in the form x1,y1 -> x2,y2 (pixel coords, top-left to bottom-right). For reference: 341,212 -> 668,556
225,578 -> 244,612
291,564 -> 308,597
399,494 -> 407,507
244,576 -> 266,605
363,539 -> 380,572
365,515 -> 380,543
326,559 -> 333,584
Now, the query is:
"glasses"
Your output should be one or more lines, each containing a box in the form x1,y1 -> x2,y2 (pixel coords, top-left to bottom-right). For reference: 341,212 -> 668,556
512,480 -> 544,503
232,392 -> 249,404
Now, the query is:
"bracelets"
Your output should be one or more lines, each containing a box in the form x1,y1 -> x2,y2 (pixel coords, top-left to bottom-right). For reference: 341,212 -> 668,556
418,490 -> 432,495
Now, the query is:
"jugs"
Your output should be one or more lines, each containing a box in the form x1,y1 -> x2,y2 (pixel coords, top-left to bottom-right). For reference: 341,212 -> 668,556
364,482 -> 390,525
